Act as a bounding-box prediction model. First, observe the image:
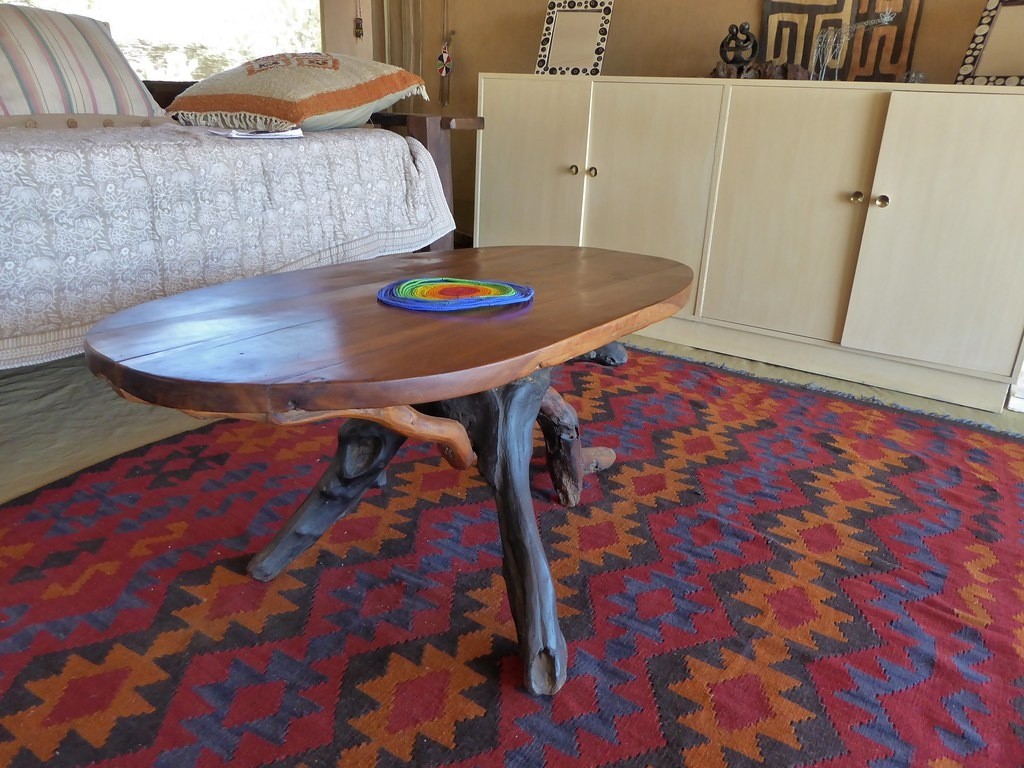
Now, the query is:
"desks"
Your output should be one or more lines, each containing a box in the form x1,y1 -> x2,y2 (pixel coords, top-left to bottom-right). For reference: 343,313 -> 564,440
365,111 -> 486,251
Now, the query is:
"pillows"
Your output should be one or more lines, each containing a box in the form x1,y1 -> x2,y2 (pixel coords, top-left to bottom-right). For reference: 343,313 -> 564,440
163,52 -> 430,131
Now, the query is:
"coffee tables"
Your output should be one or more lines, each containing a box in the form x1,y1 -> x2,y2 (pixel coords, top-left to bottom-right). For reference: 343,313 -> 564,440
89,243 -> 695,697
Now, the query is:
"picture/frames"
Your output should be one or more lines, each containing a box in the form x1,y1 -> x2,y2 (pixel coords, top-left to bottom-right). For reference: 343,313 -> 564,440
955,0 -> 1024,87
534,0 -> 615,77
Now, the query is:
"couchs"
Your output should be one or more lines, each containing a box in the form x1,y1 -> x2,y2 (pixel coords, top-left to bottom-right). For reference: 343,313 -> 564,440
0,4 -> 458,503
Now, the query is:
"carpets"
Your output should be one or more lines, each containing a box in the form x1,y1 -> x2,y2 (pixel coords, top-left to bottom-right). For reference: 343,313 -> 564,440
0,344 -> 1024,768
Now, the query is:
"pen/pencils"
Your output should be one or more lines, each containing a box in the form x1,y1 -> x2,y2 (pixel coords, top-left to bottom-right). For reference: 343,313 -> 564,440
249,130 -> 287,134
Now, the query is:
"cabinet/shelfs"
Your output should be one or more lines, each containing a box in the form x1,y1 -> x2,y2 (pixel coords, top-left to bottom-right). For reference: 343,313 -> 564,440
472,72 -> 1024,436
